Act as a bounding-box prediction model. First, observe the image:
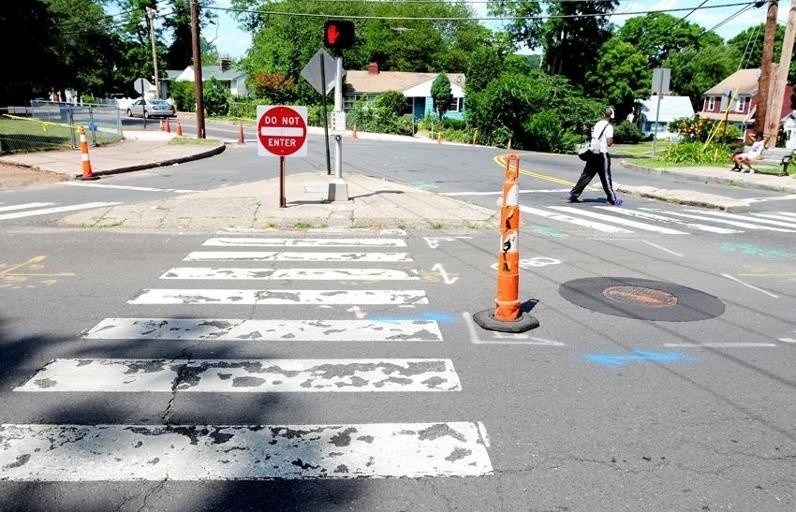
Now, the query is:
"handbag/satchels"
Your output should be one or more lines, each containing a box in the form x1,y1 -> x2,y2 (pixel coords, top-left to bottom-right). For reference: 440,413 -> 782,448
577,140 -> 602,160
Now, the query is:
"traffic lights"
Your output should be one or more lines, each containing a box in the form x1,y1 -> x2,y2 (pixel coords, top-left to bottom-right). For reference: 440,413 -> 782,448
324,20 -> 354,49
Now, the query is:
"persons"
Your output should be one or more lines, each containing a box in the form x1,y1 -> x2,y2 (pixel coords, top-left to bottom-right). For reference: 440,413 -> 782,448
565,107 -> 624,206
733,130 -> 772,173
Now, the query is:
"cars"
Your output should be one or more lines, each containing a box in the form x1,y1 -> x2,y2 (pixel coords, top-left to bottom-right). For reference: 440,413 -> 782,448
30,97 -> 46,106
126,96 -> 177,118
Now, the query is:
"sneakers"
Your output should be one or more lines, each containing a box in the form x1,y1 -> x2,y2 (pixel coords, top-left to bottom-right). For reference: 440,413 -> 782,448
565,194 -> 581,203
608,197 -> 624,208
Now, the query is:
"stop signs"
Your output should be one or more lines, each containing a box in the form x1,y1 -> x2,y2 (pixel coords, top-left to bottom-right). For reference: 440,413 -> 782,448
257,105 -> 307,156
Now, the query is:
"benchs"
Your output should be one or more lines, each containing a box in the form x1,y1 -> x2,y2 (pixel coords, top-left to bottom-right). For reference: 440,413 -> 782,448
732,145 -> 796,176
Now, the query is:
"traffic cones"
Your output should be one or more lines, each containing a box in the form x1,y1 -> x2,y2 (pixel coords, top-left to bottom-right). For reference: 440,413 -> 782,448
176,121 -> 184,136
157,117 -> 166,131
75,124 -> 102,182
237,125 -> 245,144
164,116 -> 172,133
